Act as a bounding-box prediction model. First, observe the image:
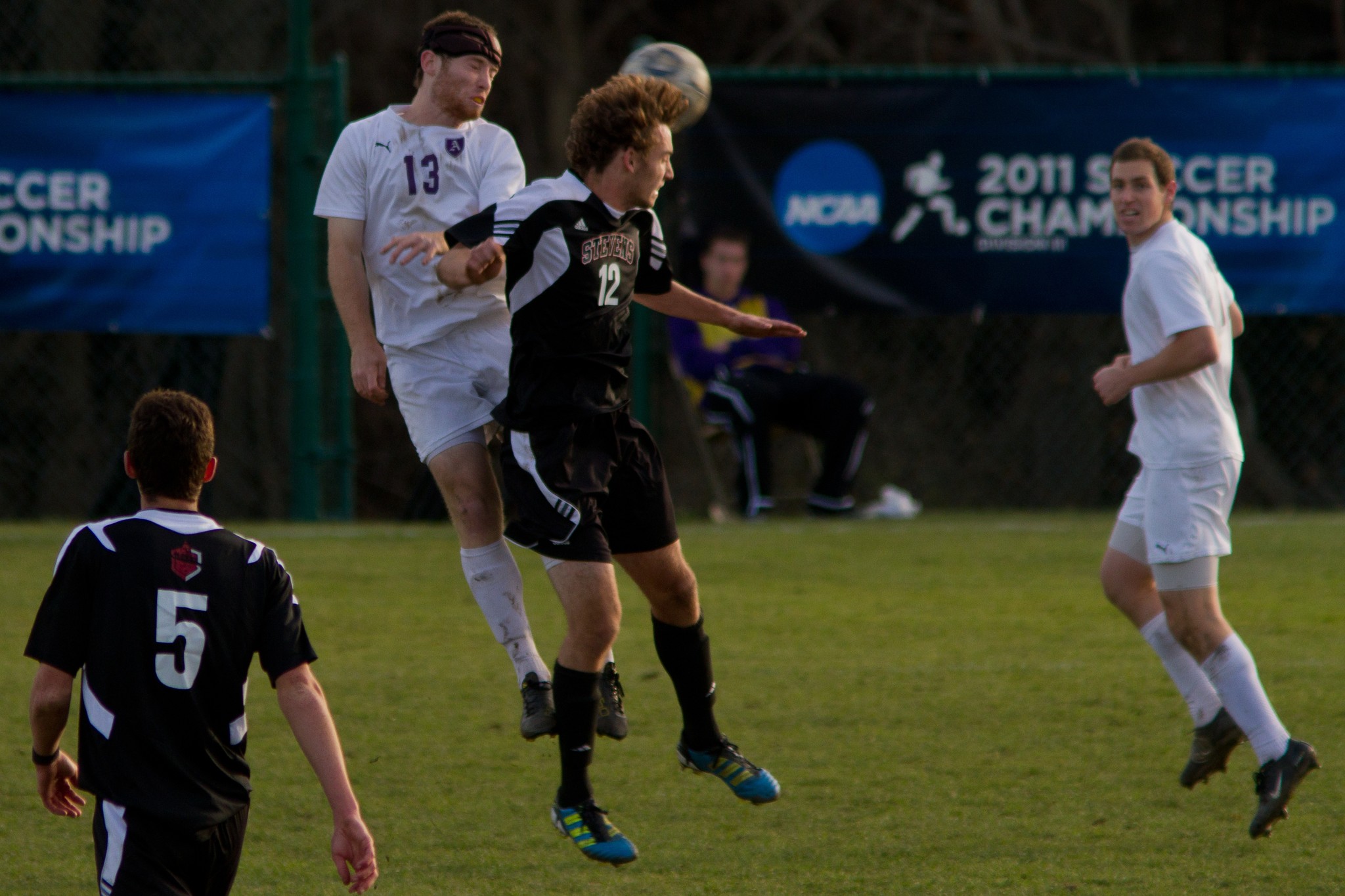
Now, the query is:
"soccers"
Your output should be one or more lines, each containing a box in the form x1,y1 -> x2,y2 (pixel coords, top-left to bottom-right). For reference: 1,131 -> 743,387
619,39 -> 712,134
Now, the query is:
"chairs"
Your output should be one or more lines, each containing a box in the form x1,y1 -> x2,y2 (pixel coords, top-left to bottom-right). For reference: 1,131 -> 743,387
662,316 -> 835,522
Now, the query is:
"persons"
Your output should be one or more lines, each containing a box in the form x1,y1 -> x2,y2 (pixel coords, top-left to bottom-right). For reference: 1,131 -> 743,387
314,9 -> 629,747
1088,136 -> 1324,842
24,394 -> 380,896
664,223 -> 881,527
432,72 -> 809,872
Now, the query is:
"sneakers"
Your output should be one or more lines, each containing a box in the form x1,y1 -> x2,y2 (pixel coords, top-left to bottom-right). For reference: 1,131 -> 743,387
1179,708 -> 1243,792
1250,739 -> 1320,839
676,735 -> 781,806
516,671 -> 557,740
587,664 -> 628,742
550,789 -> 637,869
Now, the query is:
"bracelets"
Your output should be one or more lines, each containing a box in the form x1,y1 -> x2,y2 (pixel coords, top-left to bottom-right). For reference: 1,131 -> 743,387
32,745 -> 60,764
